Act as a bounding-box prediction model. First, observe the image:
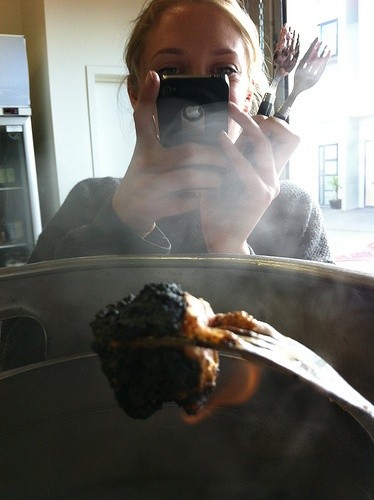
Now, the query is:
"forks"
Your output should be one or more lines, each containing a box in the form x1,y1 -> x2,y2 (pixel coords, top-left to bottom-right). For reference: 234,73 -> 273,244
275,37 -> 332,119
119,319 -> 374,449
241,23 -> 302,164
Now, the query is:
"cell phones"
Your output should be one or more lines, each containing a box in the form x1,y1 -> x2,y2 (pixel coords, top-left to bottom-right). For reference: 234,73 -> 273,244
156,74 -> 230,193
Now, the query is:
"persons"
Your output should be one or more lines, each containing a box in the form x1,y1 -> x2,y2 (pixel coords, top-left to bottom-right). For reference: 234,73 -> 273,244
26,0 -> 337,265
